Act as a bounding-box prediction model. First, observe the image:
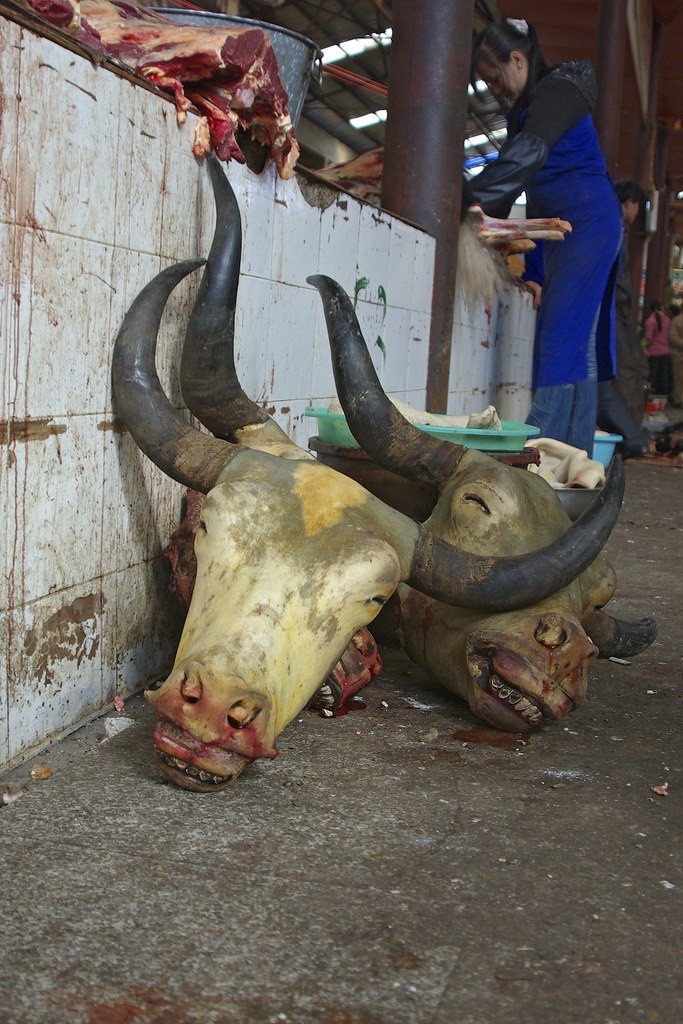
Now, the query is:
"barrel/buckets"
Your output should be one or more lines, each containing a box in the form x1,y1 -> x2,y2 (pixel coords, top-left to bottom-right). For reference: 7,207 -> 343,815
145,8 -> 321,153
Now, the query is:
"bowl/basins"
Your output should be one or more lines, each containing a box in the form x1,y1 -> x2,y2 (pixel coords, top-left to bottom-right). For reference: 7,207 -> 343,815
547,486 -> 601,522
591,430 -> 624,467
304,409 -> 541,452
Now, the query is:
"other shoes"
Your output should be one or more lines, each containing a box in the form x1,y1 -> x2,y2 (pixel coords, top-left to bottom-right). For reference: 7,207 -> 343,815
620,447 -> 648,458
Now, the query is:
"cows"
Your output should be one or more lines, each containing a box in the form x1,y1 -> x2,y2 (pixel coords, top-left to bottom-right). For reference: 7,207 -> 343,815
110,149 -> 656,792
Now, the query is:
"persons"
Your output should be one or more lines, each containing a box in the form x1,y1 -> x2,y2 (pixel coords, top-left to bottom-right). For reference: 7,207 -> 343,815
461,18 -> 623,466
596,181 -> 654,461
644,299 -> 683,415
